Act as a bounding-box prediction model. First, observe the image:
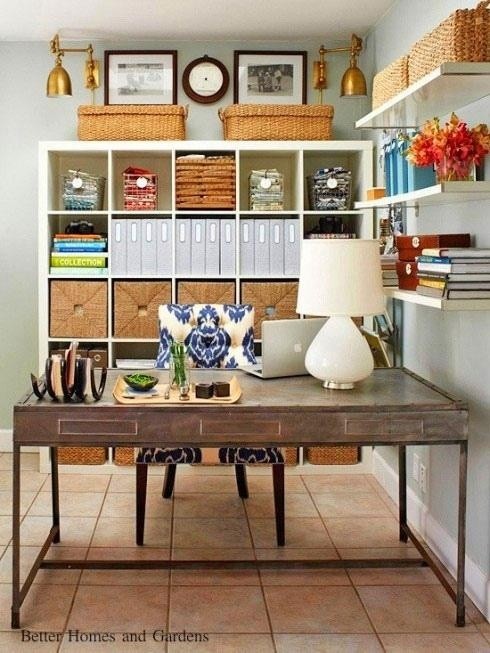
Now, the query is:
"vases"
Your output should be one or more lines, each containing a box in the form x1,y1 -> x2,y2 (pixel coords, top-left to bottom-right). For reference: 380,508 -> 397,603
436,159 -> 476,183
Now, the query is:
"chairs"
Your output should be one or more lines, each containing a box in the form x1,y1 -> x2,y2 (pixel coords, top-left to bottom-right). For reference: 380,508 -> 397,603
134,304 -> 285,546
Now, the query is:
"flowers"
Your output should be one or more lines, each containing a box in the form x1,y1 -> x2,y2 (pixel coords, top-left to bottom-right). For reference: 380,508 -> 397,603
398,111 -> 490,181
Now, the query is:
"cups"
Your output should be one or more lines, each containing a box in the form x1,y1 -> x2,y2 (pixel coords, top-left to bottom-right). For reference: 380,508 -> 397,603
194,380 -> 214,397
212,380 -> 231,398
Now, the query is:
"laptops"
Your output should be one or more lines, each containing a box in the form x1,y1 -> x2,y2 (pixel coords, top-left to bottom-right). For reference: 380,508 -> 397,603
237,316 -> 329,380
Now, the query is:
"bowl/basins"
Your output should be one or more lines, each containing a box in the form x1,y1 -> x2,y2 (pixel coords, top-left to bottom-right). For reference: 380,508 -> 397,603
365,186 -> 387,199
123,371 -> 159,390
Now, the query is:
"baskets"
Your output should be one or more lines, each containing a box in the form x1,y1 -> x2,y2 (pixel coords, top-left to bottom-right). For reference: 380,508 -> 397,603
49,280 -> 107,338
285,447 -> 298,466
217,103 -> 335,140
122,172 -> 160,210
62,175 -> 106,210
307,174 -> 353,210
241,281 -> 299,339
307,446 -> 359,465
76,103 -> 187,140
248,175 -> 287,211
113,281 -> 172,338
371,8 -> 490,112
114,446 -> 134,466
177,281 -> 235,305
57,447 -> 107,465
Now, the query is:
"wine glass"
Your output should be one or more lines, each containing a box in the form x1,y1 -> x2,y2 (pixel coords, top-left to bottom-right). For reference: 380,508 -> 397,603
178,385 -> 190,400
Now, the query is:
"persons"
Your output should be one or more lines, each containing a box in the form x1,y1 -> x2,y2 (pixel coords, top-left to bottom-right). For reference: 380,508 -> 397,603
258,70 -> 265,92
265,72 -> 272,91
275,68 -> 282,90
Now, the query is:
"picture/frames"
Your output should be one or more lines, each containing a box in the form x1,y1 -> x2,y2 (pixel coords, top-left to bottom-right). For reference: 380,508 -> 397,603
104,49 -> 177,106
234,50 -> 307,105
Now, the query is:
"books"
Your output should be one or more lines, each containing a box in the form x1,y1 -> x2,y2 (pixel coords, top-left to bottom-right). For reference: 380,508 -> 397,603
415,247 -> 490,299
381,254 -> 399,288
50,234 -> 108,269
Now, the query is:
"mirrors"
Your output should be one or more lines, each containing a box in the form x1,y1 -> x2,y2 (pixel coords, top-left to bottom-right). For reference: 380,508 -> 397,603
182,54 -> 230,104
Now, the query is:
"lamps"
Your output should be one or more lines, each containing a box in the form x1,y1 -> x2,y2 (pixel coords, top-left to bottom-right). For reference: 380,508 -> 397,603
46,33 -> 100,104
296,239 -> 385,389
313,34 -> 368,105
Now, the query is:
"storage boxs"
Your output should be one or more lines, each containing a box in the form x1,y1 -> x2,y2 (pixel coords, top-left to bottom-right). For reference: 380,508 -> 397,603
396,234 -> 470,261
395,260 -> 419,291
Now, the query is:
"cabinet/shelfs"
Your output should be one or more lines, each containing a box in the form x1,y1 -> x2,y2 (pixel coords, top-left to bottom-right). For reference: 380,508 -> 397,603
354,63 -> 490,310
38,141 -> 374,474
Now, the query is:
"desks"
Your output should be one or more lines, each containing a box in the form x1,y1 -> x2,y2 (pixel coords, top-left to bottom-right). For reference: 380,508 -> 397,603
12,369 -> 470,629
